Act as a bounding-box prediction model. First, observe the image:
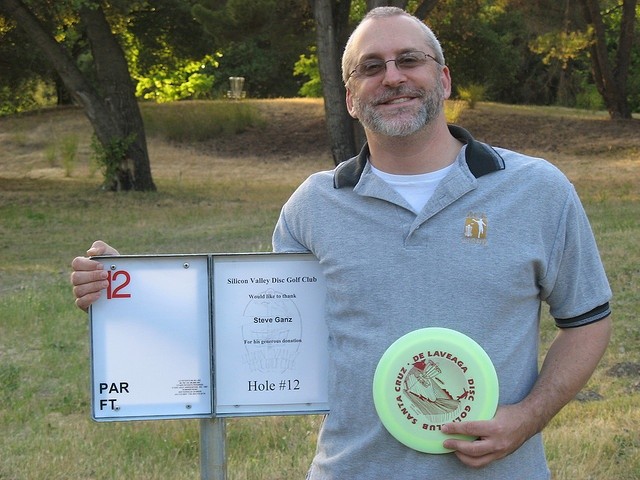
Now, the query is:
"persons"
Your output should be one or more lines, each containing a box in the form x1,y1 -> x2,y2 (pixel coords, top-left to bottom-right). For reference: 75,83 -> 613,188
70,7 -> 613,480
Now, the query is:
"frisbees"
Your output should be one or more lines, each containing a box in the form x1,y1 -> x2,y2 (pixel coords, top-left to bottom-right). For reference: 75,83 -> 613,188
372,327 -> 500,455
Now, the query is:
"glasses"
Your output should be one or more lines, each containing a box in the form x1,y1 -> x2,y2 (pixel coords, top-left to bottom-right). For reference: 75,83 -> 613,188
345,49 -> 443,83
